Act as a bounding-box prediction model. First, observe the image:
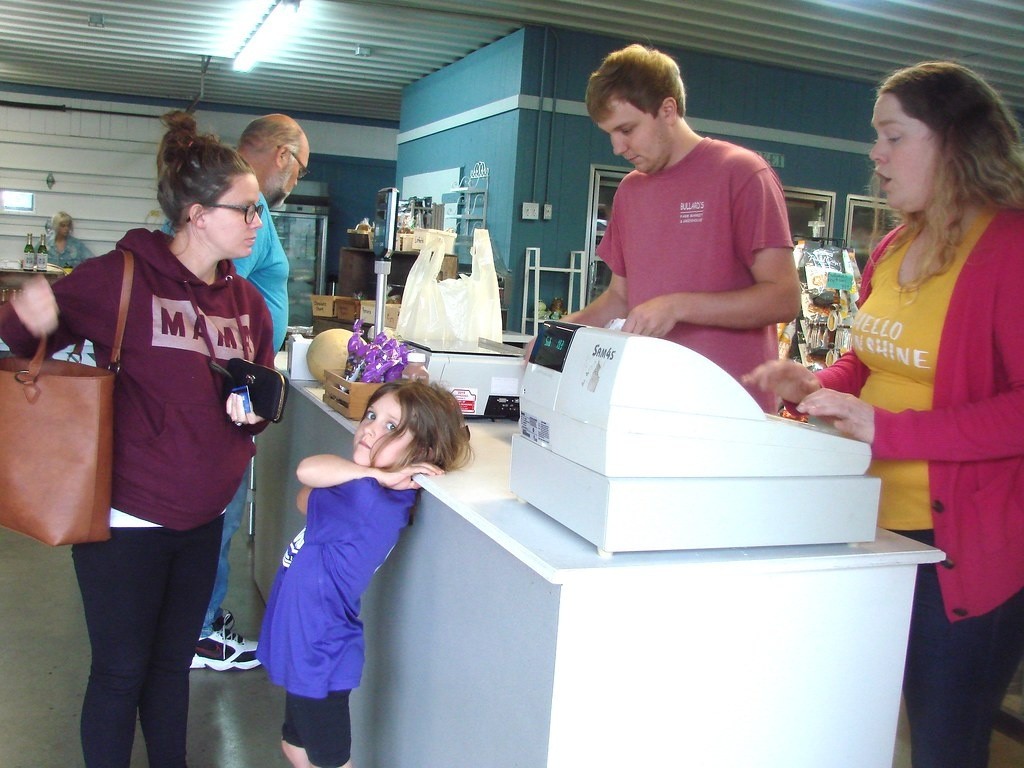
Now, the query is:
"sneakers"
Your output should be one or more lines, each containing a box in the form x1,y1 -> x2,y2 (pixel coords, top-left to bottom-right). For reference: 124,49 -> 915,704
188,609 -> 263,672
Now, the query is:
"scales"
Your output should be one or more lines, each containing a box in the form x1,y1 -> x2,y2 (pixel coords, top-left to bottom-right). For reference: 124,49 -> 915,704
373,186 -> 527,423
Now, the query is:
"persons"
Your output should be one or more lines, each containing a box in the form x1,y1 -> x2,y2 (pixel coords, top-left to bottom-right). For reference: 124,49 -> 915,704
1,104 -> 274,768
254,378 -> 473,768
20,212 -> 97,269
159,114 -> 308,673
524,42 -> 804,416
742,59 -> 1024,768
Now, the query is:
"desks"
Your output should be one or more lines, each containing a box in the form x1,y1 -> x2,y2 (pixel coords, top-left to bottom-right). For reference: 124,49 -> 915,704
500,329 -> 535,344
0,268 -> 65,291
338,247 -> 458,303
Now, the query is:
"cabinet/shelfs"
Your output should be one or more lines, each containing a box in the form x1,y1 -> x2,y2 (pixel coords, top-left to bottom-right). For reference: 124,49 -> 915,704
444,161 -> 490,246
521,245 -> 586,338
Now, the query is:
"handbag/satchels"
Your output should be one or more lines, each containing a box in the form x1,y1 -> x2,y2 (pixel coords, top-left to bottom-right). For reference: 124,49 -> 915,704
0,246 -> 135,549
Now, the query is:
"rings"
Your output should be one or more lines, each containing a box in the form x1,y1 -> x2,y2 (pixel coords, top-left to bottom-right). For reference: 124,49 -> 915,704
235,422 -> 242,426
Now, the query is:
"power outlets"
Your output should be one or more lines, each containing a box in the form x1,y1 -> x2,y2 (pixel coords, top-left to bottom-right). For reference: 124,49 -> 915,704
522,201 -> 540,221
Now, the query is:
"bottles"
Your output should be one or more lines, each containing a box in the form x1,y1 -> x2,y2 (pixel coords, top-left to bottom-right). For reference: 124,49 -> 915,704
37,233 -> 48,272
23,232 -> 35,271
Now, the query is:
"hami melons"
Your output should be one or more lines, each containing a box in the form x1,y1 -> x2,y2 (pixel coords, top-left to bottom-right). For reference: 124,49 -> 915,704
307,328 -> 368,385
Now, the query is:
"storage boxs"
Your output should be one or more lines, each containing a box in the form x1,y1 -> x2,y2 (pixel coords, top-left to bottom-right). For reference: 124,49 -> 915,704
360,301 -> 376,323
321,368 -> 385,421
412,226 -> 457,255
311,295 -> 351,318
385,304 -> 400,327
336,298 -> 359,324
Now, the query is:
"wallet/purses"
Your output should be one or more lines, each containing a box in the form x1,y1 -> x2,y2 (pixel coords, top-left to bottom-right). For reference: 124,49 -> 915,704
210,357 -> 289,424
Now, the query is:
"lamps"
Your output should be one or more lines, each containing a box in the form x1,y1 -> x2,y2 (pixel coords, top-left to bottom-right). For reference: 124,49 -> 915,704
47,171 -> 55,188
232,0 -> 300,73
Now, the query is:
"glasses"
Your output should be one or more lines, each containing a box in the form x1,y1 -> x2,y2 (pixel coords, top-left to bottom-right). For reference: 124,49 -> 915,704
276,145 -> 311,180
185,201 -> 264,224
